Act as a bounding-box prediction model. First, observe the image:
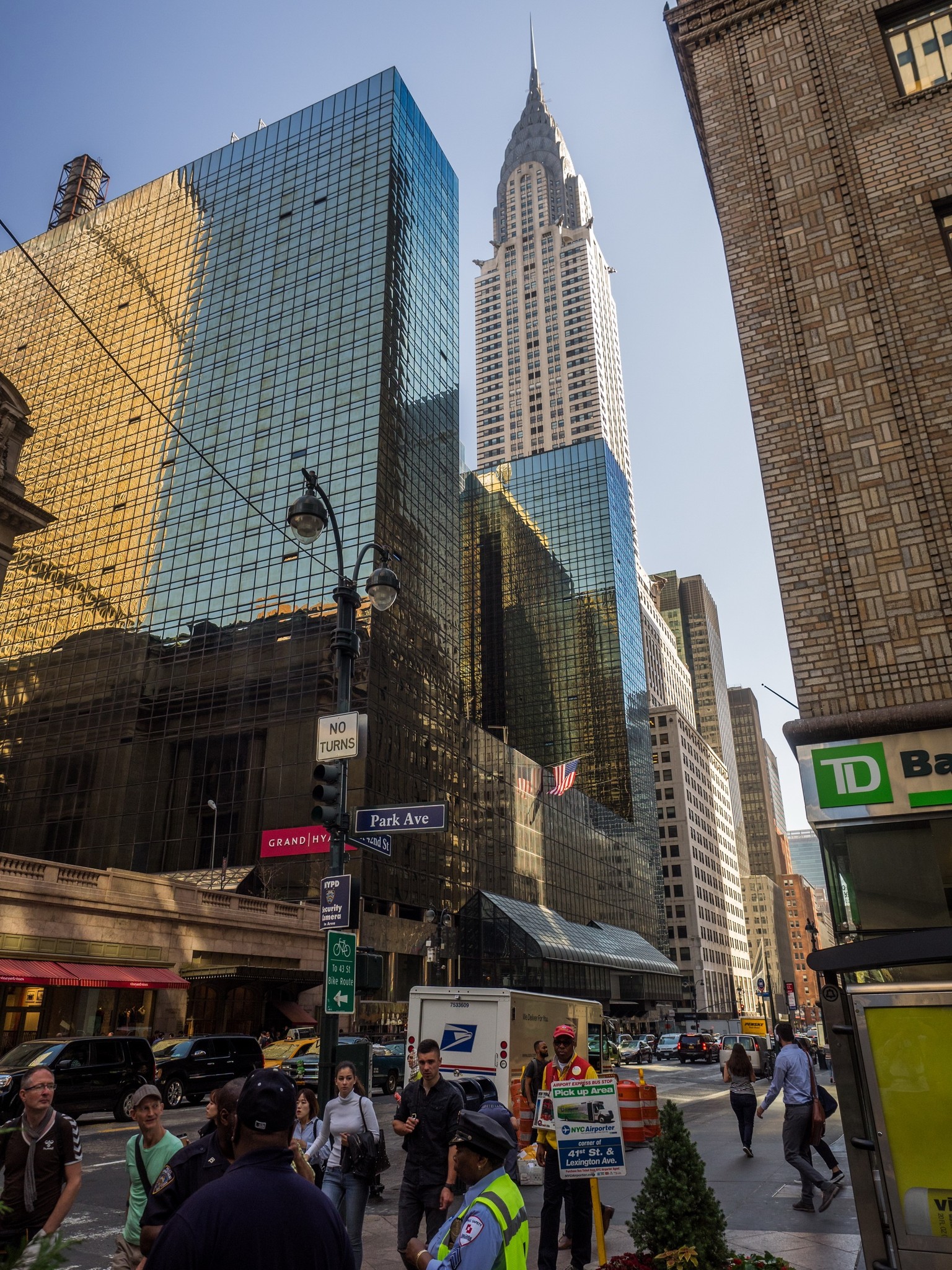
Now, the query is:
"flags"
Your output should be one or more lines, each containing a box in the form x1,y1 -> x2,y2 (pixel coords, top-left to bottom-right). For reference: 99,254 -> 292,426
517,765 -> 542,798
546,761 -> 577,796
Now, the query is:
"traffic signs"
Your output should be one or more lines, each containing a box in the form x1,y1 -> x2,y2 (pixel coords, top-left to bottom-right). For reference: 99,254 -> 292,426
324,929 -> 358,1015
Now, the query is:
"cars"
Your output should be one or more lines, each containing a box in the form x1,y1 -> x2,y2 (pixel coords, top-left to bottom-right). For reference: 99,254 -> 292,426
588,1034 -> 609,1041
260,1027 -> 320,1073
617,1040 -> 653,1065
631,1035 -> 658,1056
607,1034 -> 634,1050
769,1026 -> 819,1067
383,1043 -> 405,1056
656,1033 -> 683,1060
586,1040 -> 622,1071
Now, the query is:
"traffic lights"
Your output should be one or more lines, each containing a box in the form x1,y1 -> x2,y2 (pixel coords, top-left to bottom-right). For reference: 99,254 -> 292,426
313,762 -> 342,831
741,1005 -> 744,1011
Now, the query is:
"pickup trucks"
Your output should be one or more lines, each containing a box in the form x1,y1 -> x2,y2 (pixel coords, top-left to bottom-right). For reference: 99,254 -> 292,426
281,1036 -> 406,1096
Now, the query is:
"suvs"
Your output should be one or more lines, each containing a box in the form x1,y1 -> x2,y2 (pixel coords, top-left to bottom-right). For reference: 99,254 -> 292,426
0,1034 -> 156,1128
678,1033 -> 720,1064
150,1032 -> 266,1109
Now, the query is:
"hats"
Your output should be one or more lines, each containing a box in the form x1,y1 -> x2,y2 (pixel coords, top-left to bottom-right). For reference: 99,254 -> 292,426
449,1109 -> 516,1161
132,1084 -> 163,1109
238,1067 -> 298,1135
553,1025 -> 575,1040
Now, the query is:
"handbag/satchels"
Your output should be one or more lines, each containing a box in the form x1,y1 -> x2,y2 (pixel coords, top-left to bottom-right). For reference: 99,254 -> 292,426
816,1085 -> 839,1118
358,1096 -> 390,1177
812,1098 -> 827,1146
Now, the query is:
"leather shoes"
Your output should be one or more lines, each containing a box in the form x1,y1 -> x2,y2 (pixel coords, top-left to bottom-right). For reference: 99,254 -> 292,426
558,1230 -> 571,1250
604,1205 -> 615,1235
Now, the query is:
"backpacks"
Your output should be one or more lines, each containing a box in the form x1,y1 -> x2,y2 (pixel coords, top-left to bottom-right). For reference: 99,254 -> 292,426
521,1059 -> 538,1097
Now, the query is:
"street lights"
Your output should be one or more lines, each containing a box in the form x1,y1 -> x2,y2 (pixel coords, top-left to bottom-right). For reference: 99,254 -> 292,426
290,466 -> 406,1121
737,984 -> 744,1016
208,798 -> 220,893
803,917 -> 830,1046
682,979 -> 705,1033
423,903 -> 453,985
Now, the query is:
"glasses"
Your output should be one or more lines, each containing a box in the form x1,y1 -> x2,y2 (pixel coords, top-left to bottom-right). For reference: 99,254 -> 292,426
554,1039 -> 575,1047
24,1083 -> 57,1091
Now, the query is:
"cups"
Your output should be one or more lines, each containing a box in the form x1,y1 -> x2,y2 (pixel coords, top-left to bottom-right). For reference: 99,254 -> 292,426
176,1133 -> 188,1147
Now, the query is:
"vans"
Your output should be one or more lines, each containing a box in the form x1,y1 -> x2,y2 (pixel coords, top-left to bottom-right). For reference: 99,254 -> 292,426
718,1034 -> 770,1079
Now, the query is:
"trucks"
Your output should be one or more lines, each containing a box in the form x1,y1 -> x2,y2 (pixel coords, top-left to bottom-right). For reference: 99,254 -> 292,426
404,985 -> 620,1129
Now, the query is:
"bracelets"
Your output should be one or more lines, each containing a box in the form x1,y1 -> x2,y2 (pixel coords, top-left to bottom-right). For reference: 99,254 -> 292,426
415,1250 -> 428,1270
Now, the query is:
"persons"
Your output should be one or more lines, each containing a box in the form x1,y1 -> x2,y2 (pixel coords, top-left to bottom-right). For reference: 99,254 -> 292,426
109,1024 -> 614,1270
0,1065 -> 83,1270
756,1023 -> 845,1213
724,1043 -> 757,1157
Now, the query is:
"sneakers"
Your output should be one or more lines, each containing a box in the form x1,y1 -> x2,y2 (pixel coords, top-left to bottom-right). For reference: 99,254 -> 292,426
792,1202 -> 815,1212
818,1185 -> 840,1212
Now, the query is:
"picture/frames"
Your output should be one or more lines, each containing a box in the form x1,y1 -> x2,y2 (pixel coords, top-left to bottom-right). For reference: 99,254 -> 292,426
35,989 -> 44,1003
25,990 -> 36,1002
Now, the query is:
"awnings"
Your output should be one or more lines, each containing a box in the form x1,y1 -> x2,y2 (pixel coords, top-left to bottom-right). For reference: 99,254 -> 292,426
272,1001 -> 318,1024
0,958 -> 191,989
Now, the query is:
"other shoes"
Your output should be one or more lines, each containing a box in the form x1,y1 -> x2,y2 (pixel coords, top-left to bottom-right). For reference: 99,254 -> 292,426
564,1263 -> 577,1270
794,1178 -> 814,1185
830,1171 -> 845,1183
743,1147 -> 753,1158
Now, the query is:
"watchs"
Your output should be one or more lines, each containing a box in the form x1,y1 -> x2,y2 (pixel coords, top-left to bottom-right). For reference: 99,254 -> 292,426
444,1183 -> 456,1193
